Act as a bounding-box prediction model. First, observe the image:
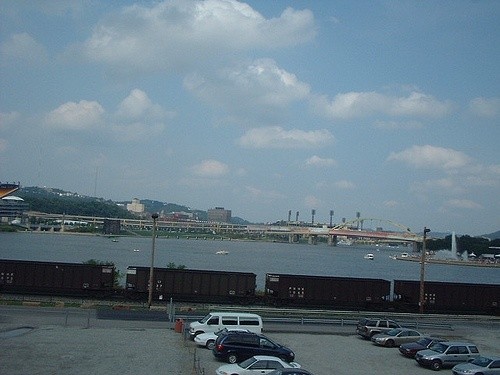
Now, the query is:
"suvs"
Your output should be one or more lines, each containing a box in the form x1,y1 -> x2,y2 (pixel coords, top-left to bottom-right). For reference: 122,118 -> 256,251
357,316 -> 403,339
212,329 -> 296,365
414,341 -> 481,372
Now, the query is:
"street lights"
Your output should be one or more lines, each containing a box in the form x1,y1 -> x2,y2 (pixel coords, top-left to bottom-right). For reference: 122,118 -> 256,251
146,212 -> 159,307
419,226 -> 431,314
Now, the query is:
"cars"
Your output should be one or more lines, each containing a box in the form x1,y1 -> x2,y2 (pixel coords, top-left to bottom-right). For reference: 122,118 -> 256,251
451,356 -> 500,375
371,327 -> 430,348
215,354 -> 302,375
399,338 -> 449,359
265,368 -> 313,375
193,327 -> 253,351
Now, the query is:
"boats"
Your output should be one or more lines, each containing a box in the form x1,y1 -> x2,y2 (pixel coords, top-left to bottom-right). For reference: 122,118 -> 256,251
364,254 -> 374,260
216,250 -> 229,255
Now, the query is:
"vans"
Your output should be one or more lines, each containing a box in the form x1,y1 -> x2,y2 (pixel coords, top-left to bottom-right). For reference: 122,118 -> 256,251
188,312 -> 264,339
